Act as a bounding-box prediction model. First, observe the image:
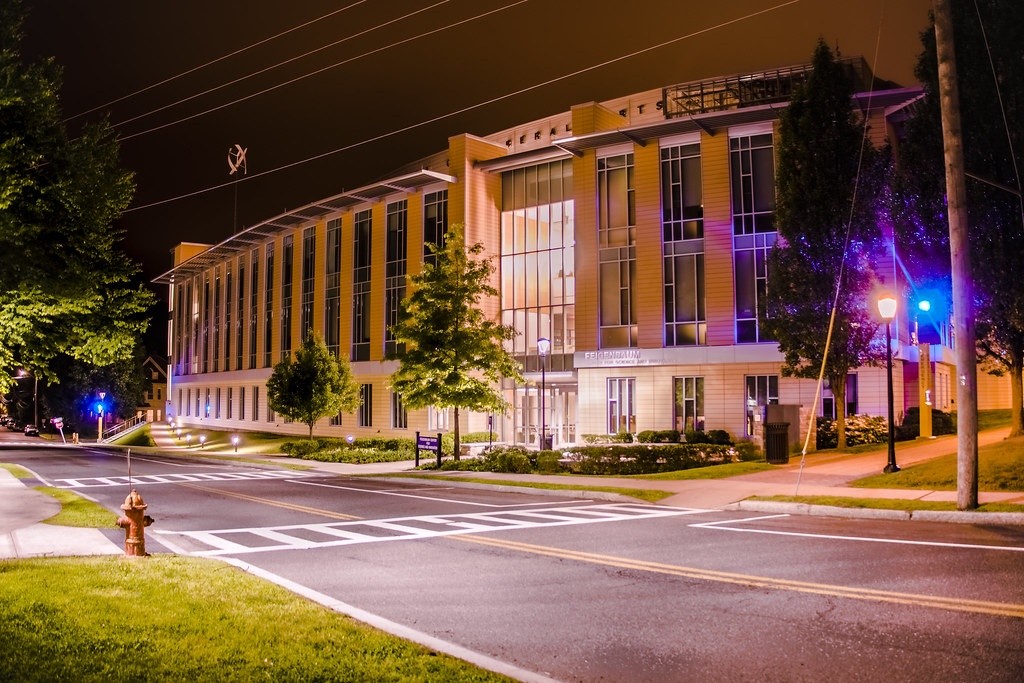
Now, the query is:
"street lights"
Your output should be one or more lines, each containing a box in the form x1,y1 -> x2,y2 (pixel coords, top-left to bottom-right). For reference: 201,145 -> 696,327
537,339 -> 551,452
20,371 -> 37,428
878,294 -> 900,473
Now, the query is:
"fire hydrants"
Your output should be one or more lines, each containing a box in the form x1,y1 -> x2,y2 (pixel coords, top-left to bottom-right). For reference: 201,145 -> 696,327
116,489 -> 154,556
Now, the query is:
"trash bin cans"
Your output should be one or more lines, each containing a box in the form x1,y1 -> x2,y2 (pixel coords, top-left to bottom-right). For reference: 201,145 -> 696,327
762,422 -> 791,465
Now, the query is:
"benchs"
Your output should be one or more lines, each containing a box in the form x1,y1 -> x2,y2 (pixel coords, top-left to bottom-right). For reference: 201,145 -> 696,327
461,442 -> 507,456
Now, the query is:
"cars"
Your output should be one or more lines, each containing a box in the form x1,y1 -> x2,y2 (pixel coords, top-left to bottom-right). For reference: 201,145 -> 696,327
6,419 -> 24,432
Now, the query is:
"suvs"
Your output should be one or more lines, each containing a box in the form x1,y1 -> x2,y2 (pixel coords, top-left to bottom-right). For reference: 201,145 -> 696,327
0,415 -> 12,426
25,425 -> 39,436
